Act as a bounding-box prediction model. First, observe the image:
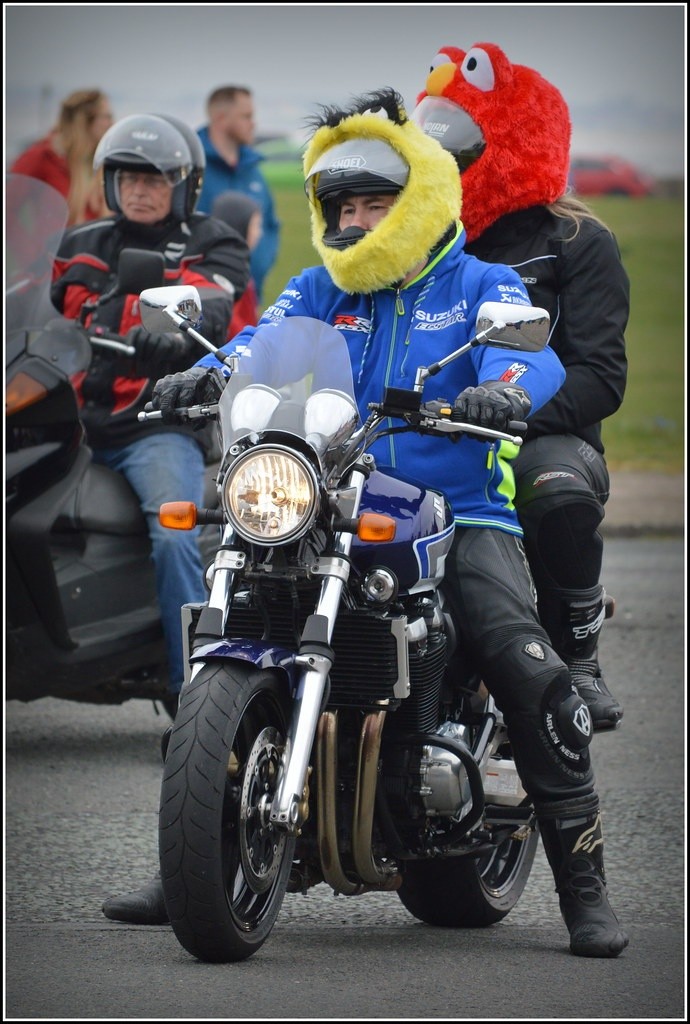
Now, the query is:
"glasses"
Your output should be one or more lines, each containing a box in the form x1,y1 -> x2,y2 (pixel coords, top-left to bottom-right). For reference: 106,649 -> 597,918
118,172 -> 175,189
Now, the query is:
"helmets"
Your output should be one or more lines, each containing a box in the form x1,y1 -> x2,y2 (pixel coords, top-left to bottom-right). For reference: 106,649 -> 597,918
103,113 -> 205,222
312,137 -> 457,252
423,108 -> 546,232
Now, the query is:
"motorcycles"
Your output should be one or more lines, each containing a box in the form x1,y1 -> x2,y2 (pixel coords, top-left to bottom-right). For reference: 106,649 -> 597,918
3,172 -> 257,728
137,285 -> 617,967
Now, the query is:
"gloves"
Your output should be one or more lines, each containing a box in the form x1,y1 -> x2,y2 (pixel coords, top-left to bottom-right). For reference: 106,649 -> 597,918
152,366 -> 226,432
126,326 -> 182,379
448,381 -> 532,444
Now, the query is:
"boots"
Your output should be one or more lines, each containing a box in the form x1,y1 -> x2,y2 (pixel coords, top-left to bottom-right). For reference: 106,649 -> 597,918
535,792 -> 625,958
536,583 -> 623,727
101,778 -> 258,925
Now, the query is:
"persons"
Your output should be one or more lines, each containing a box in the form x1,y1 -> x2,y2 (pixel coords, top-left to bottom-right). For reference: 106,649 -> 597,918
50,112 -> 250,764
102,43 -> 639,955
5,90 -> 118,270
